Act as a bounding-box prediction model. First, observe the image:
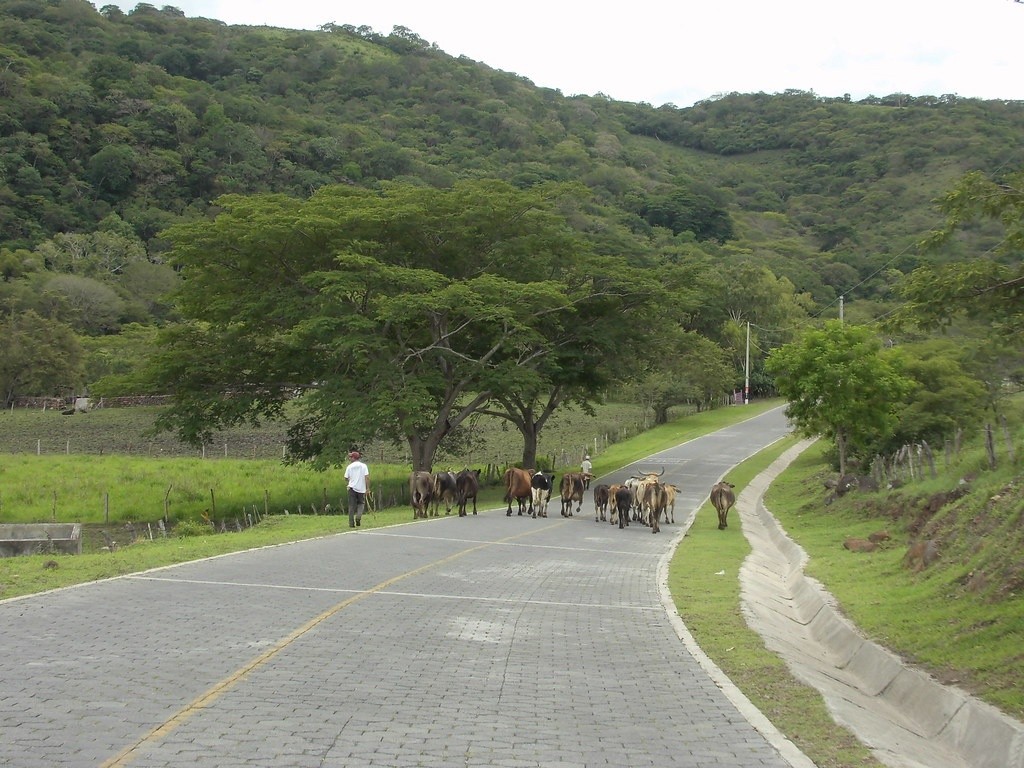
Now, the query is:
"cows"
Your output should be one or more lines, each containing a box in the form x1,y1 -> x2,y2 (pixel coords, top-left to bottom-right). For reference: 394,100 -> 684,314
594,465 -> 681,534
559,472 -> 596,517
411,468 -> 481,519
710,482 -> 735,530
504,467 -> 555,517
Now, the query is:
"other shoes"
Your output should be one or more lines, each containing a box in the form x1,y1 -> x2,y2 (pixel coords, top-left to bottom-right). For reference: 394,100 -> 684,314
356,519 -> 361,526
587,485 -> 589,490
350,524 -> 355,527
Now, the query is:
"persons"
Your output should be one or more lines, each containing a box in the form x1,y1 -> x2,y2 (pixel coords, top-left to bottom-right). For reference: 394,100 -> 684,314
344,453 -> 369,527
581,456 -> 592,490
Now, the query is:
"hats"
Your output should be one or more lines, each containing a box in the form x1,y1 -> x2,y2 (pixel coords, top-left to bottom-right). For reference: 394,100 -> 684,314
586,455 -> 590,459
349,452 -> 360,459
